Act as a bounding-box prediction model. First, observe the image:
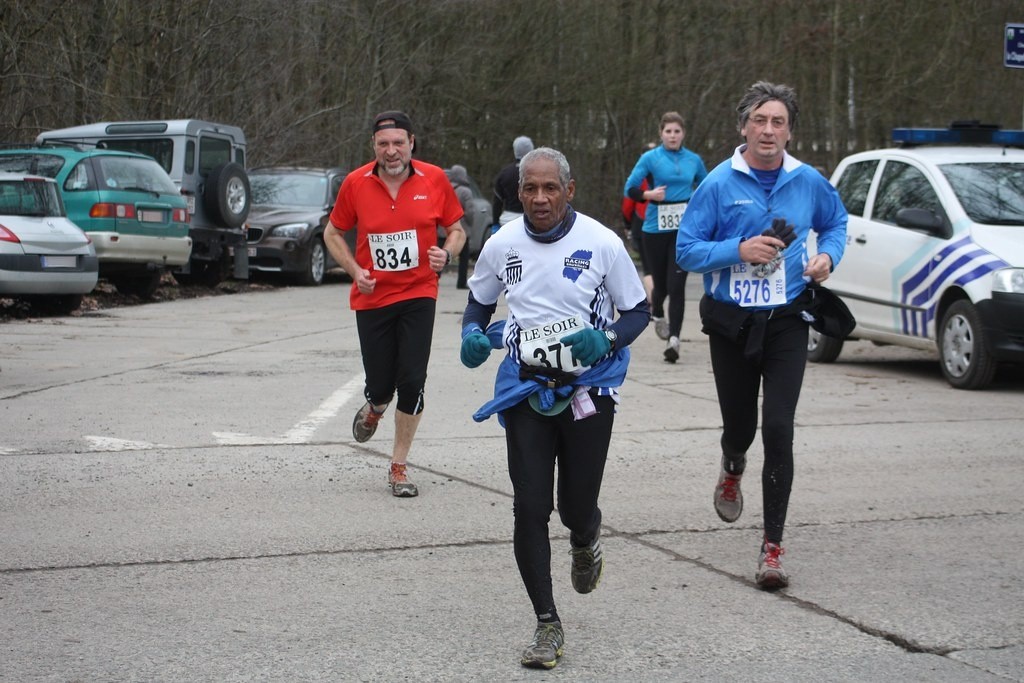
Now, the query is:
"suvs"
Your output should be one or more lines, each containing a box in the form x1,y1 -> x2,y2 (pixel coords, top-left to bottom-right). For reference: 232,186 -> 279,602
0,143 -> 193,297
34,119 -> 251,287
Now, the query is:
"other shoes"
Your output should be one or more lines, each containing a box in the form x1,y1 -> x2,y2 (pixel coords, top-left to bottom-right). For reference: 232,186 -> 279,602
458,284 -> 470,289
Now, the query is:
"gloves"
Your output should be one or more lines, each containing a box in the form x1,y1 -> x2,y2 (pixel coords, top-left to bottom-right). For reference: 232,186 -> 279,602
560,327 -> 611,367
750,218 -> 797,266
460,332 -> 491,368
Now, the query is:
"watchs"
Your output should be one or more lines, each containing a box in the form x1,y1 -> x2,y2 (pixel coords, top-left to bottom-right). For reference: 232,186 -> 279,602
603,328 -> 617,347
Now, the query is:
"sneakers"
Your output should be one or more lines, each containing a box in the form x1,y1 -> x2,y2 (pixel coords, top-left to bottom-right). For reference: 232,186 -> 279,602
713,453 -> 747,523
353,401 -> 389,443
568,524 -> 604,594
664,335 -> 680,363
388,462 -> 418,497
520,621 -> 564,669
755,535 -> 789,588
652,316 -> 670,340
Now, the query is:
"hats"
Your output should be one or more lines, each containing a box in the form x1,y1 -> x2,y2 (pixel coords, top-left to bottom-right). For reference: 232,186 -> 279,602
513,137 -> 534,159
372,110 -> 416,153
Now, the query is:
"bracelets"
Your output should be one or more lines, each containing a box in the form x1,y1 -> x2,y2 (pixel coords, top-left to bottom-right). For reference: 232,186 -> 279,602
443,249 -> 452,265
436,263 -> 440,268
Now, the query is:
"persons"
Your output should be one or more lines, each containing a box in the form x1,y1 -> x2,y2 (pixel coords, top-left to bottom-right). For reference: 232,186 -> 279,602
676,79 -> 849,586
437,165 -> 475,290
622,142 -> 654,320
624,111 -> 708,363
460,146 -> 652,669
491,135 -> 534,235
324,109 -> 467,497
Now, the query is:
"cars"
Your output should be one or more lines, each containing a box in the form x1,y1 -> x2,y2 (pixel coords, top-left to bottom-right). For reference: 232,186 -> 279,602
439,170 -> 494,261
806,121 -> 1024,389
0,170 -> 98,312
230,165 -> 358,286
